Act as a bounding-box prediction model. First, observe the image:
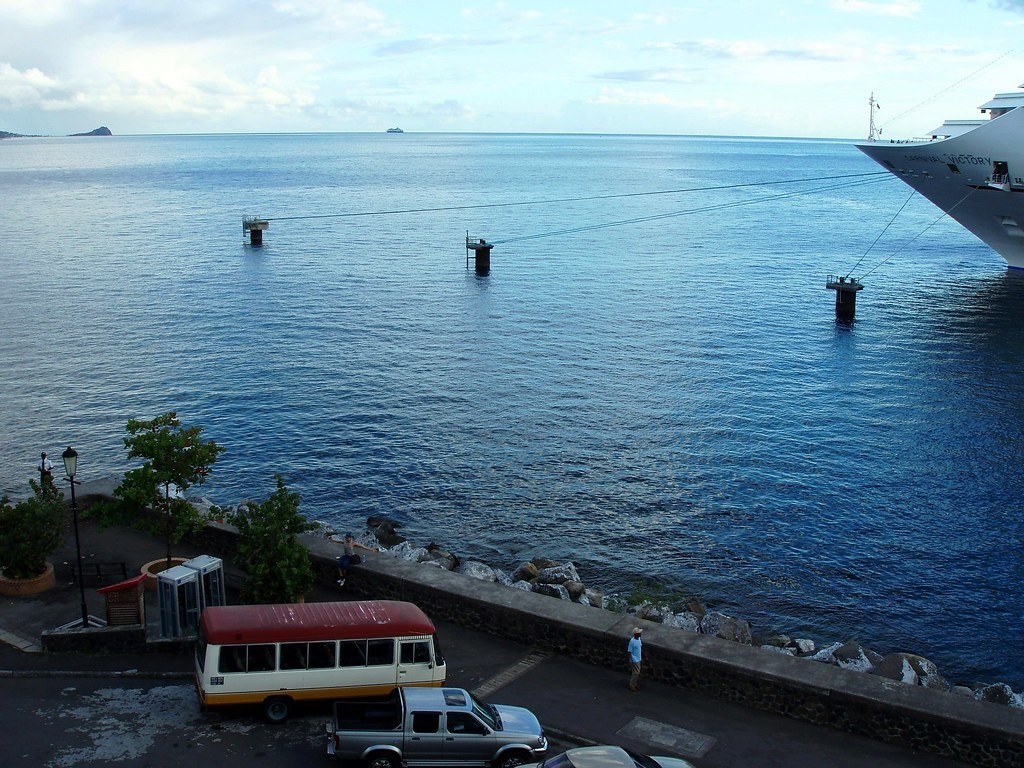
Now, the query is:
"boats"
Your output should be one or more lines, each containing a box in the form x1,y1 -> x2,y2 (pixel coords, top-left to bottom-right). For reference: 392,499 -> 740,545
387,127 -> 403,133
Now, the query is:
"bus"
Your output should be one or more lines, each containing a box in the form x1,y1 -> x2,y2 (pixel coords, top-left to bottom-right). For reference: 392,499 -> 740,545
196,599 -> 448,724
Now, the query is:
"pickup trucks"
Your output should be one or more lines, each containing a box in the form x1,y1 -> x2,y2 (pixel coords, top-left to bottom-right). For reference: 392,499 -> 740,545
324,686 -> 549,768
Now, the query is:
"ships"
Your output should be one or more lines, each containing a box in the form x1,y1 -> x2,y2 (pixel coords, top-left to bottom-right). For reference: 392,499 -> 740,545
854,81 -> 1024,269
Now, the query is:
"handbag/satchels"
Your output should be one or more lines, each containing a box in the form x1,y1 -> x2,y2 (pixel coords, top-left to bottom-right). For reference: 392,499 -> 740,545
348,554 -> 361,564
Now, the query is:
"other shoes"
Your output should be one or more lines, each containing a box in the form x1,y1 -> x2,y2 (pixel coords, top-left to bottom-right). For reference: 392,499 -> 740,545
337,579 -> 345,586
630,685 -> 640,691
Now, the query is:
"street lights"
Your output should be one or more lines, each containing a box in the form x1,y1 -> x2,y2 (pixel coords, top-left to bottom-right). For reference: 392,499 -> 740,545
61,446 -> 90,626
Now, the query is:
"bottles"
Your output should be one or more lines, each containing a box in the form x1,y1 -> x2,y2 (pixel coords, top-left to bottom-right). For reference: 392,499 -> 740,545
363,554 -> 365,562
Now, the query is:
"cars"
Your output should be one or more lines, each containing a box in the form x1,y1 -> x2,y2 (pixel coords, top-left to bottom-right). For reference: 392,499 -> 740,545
517,745 -> 694,768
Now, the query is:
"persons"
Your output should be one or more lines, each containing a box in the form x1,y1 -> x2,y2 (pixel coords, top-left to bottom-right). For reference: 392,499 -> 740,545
329,532 -> 376,586
37,452 -> 57,496
628,627 -> 644,692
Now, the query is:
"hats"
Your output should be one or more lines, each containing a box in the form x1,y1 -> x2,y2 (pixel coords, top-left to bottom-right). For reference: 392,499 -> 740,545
345,533 -> 351,538
633,628 -> 643,634
